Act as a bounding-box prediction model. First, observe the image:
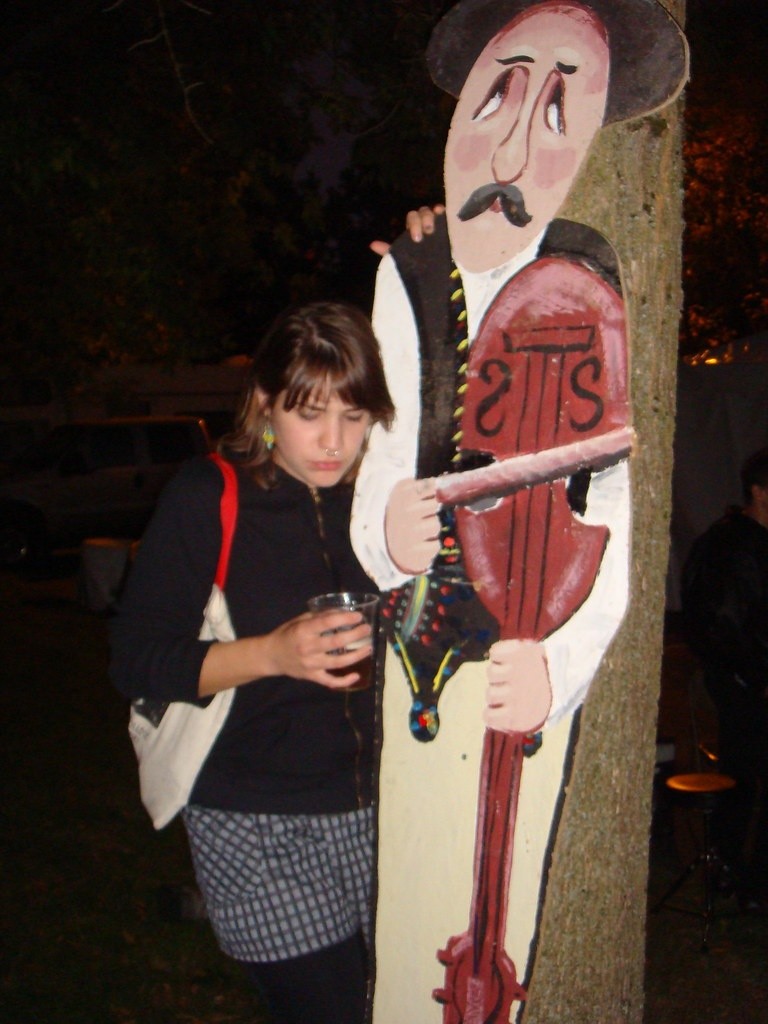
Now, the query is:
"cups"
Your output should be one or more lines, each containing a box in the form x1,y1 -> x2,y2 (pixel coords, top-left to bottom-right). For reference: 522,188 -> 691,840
308,591 -> 380,692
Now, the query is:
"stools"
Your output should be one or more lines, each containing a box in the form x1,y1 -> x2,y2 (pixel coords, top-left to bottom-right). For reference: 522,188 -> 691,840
645,771 -> 768,942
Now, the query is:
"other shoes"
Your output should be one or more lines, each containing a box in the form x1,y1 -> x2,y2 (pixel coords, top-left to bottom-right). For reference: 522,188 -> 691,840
707,844 -> 742,881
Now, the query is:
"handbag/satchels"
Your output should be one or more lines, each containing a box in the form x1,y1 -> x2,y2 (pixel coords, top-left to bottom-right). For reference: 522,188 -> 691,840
127,453 -> 239,830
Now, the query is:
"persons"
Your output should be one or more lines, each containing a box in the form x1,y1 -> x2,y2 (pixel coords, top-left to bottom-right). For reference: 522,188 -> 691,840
107,203 -> 444,1024
695,447 -> 767,913
